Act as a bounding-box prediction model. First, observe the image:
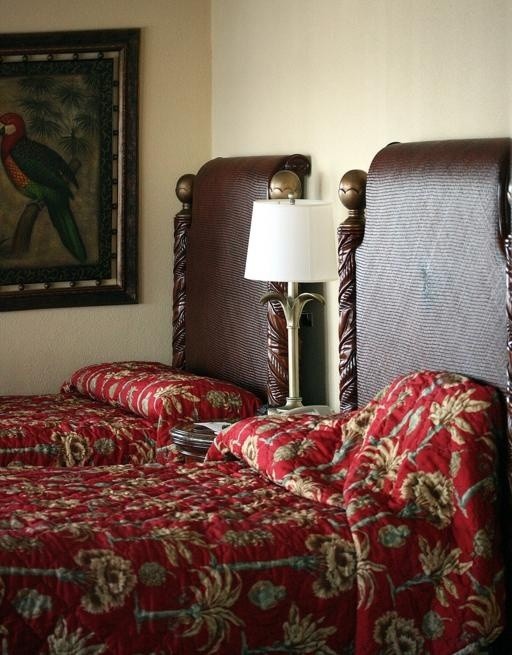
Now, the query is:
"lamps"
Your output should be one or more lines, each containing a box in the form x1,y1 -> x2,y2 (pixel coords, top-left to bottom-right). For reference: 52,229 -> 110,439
243,192 -> 339,417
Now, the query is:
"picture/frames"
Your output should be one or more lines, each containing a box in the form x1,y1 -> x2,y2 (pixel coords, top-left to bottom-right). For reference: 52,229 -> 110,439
1,27 -> 137,312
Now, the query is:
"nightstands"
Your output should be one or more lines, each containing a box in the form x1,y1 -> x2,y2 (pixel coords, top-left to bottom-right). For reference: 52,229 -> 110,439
169,419 -> 240,463
